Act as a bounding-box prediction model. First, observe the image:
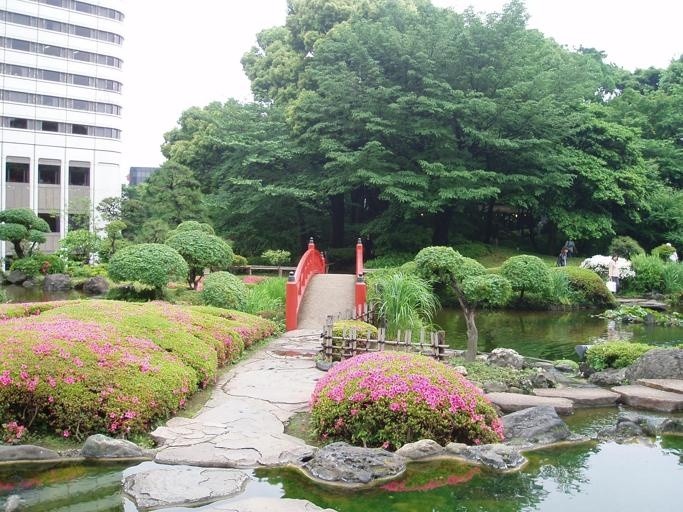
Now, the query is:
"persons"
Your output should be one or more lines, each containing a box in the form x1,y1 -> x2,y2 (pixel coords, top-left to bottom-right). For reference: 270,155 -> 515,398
557,247 -> 569,266
608,253 -> 620,293
565,235 -> 578,257
665,243 -> 678,263
